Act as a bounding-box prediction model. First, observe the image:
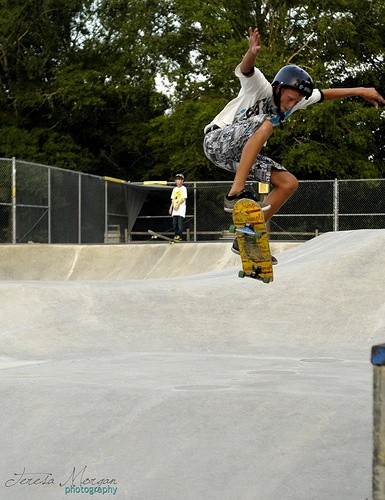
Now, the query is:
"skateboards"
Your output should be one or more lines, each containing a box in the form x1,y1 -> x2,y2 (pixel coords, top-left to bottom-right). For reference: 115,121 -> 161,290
147,229 -> 180,244
229,198 -> 273,284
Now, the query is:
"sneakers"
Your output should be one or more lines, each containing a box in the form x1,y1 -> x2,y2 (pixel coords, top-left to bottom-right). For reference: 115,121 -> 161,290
231,234 -> 280,267
223,190 -> 271,214
175,236 -> 182,239
173,236 -> 177,238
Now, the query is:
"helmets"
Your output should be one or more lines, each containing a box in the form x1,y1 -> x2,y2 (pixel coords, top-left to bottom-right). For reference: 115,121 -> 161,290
271,64 -> 313,100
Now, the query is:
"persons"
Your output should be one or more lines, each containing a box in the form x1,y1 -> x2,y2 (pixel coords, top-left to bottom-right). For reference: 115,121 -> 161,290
204,27 -> 383,265
169,174 -> 187,241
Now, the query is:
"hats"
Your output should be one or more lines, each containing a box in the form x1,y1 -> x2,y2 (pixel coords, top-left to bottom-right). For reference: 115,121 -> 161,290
175,173 -> 186,181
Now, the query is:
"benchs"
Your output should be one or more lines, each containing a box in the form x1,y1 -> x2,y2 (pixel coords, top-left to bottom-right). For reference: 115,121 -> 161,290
219,230 -> 237,239
